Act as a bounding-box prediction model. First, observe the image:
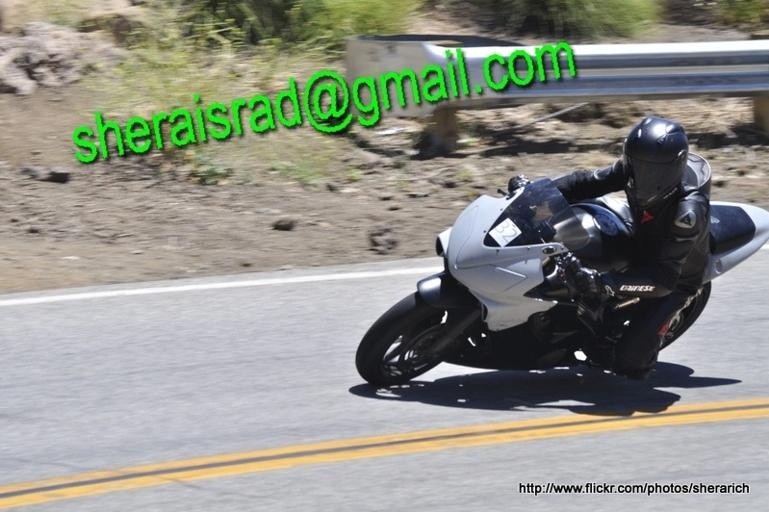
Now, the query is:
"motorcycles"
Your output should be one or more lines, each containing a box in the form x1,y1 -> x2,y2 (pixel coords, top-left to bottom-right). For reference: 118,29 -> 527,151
355,176 -> 768,388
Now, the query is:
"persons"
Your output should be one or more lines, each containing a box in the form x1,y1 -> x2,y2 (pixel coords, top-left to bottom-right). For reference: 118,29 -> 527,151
508,115 -> 713,381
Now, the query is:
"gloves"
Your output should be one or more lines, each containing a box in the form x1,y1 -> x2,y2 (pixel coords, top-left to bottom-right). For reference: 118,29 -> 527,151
567,268 -> 603,294
507,174 -> 531,192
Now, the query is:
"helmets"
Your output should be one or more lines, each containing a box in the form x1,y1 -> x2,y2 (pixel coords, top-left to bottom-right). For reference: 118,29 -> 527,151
621,115 -> 687,197
